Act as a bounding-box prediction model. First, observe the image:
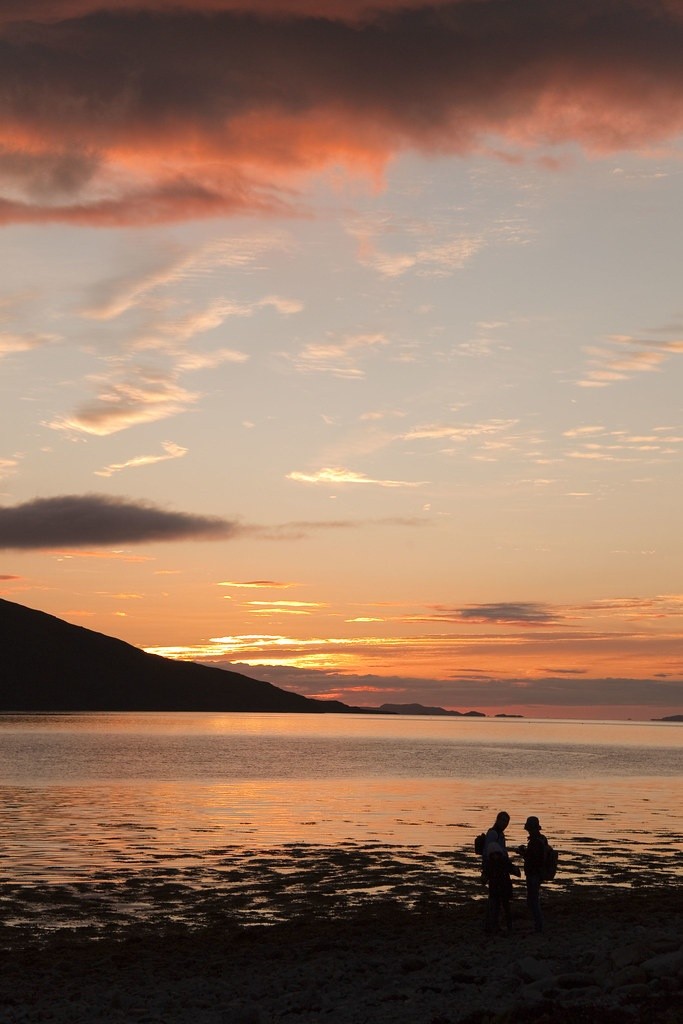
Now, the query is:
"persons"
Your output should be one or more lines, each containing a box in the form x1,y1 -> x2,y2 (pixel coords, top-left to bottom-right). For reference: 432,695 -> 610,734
516,816 -> 545,932
484,811 -> 518,929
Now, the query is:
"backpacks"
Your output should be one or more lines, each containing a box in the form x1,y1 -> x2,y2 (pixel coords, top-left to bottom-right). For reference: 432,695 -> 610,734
542,845 -> 558,880
475,833 -> 485,855
